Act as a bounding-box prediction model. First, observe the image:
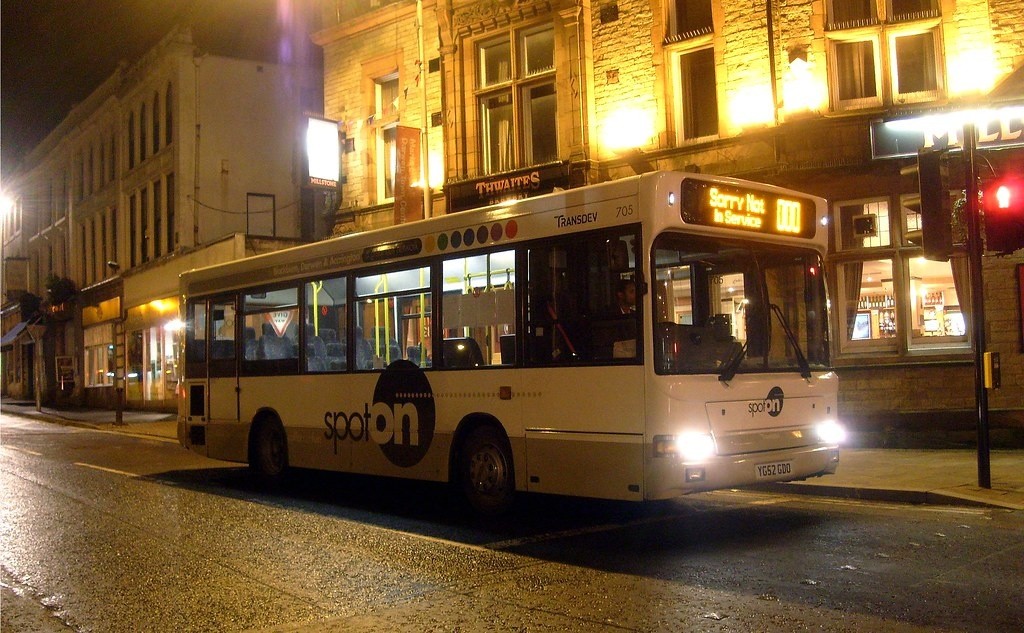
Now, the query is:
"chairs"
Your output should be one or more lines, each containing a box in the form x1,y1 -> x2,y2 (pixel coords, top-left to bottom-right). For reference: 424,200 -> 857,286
442,336 -> 484,365
245,322 -> 429,371
500,334 -> 530,364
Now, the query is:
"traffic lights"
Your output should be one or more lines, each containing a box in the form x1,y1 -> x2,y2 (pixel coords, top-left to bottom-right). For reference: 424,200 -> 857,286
987,180 -> 1022,217
900,147 -> 952,262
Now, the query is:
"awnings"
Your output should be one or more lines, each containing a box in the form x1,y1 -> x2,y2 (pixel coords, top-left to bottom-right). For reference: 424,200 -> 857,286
1,322 -> 28,347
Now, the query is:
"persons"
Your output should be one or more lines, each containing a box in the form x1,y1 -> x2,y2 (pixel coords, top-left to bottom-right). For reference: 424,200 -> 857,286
605,276 -> 636,316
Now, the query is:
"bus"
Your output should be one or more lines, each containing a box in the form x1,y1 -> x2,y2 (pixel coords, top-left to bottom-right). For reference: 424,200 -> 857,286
176,170 -> 840,529
